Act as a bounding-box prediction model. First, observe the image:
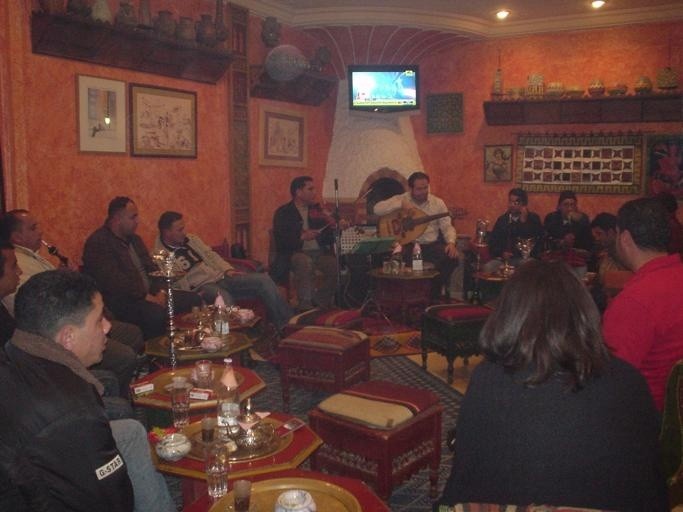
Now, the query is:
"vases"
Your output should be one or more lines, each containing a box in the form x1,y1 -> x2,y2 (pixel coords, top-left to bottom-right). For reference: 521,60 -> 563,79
262,16 -> 280,50
41,0 -> 217,47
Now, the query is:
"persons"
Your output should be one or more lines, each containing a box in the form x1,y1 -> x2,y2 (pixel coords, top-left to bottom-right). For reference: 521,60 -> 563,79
544,189 -> 592,248
492,187 -> 545,257
588,210 -> 617,252
0,238 -> 24,354
4,208 -> 145,385
485,148 -> 510,182
84,194 -> 201,332
372,171 -> 459,263
0,270 -> 175,511
431,257 -> 667,512
599,191 -> 682,434
271,175 -> 339,283
150,208 -> 298,368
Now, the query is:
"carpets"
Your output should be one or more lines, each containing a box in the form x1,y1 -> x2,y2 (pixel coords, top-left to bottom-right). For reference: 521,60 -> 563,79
124,326 -> 465,512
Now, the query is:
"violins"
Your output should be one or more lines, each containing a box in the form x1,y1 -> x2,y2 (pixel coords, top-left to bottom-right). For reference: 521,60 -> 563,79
307,202 -> 357,229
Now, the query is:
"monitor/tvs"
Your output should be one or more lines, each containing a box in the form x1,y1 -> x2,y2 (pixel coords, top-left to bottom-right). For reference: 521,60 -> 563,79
348,64 -> 420,111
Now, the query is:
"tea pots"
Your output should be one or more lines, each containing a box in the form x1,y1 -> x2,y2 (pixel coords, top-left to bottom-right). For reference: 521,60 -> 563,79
222,399 -> 274,450
156,432 -> 190,463
273,488 -> 317,512
202,336 -> 222,353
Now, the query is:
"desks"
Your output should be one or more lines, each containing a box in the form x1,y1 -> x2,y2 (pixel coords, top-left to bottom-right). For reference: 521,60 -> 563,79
151,412 -> 324,505
179,469 -> 390,512
144,331 -> 254,363
166,312 -> 262,332
368,267 -> 440,328
129,362 -> 266,420
472,257 -> 510,282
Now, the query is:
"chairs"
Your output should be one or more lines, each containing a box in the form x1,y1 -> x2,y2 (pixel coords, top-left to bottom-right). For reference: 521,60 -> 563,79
268,228 -> 319,304
166,238 -> 266,326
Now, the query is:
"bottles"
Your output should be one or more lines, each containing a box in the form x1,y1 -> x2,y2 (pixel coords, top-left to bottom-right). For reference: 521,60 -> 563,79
412,239 -> 424,272
217,360 -> 241,435
213,287 -> 229,336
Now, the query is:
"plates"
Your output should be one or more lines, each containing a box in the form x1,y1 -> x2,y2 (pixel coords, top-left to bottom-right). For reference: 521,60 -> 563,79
160,329 -> 234,353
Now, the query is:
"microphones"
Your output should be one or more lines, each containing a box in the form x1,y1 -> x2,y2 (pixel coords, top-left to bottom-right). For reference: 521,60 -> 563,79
37,237 -> 70,265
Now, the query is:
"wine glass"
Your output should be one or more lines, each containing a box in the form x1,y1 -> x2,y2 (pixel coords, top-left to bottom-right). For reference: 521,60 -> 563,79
516,236 -> 535,261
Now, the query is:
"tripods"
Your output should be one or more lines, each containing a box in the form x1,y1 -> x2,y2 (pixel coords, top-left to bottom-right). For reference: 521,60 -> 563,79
356,255 -> 393,326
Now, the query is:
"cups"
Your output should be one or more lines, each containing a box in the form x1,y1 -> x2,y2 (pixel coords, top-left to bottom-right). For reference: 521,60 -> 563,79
195,359 -> 211,391
172,386 -> 190,428
201,416 -> 215,441
380,260 -> 406,276
231,477 -> 251,511
203,445 -> 228,497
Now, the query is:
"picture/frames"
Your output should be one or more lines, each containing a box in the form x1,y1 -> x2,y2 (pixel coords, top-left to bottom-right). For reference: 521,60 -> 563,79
76,72 -> 129,155
484,144 -> 513,181
128,82 -> 197,158
258,103 -> 307,168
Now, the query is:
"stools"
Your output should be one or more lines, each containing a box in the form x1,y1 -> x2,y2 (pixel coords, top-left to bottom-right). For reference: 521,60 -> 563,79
309,381 -> 443,506
421,302 -> 495,385
314,309 -> 363,330
277,327 -> 371,413
